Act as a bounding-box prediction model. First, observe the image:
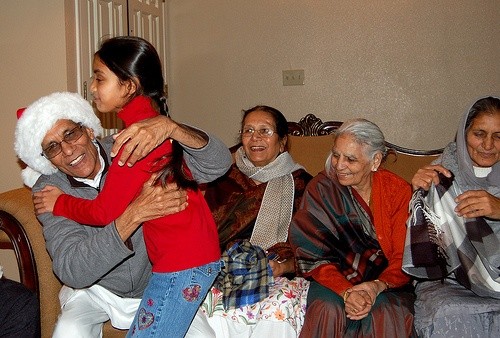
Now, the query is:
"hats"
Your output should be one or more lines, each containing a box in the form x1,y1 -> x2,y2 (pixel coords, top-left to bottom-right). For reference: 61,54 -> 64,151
13,92 -> 104,188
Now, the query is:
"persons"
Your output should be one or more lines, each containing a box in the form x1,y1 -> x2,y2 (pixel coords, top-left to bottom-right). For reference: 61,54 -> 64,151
411,95 -> 500,338
32,36 -> 221,338
203,105 -> 314,338
290,117 -> 413,338
13,109 -> 232,338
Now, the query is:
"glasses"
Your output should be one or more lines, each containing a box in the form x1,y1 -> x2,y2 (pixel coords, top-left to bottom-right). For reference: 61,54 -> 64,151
239,128 -> 278,137
41,123 -> 83,158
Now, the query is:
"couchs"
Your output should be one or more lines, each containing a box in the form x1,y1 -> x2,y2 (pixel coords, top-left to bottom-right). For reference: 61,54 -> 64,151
0,114 -> 445,338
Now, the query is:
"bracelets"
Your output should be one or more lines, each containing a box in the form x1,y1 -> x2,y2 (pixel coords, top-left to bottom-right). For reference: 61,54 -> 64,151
374,279 -> 390,292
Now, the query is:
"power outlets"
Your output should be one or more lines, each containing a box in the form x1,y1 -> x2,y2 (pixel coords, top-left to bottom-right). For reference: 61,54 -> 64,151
283,70 -> 305,85
